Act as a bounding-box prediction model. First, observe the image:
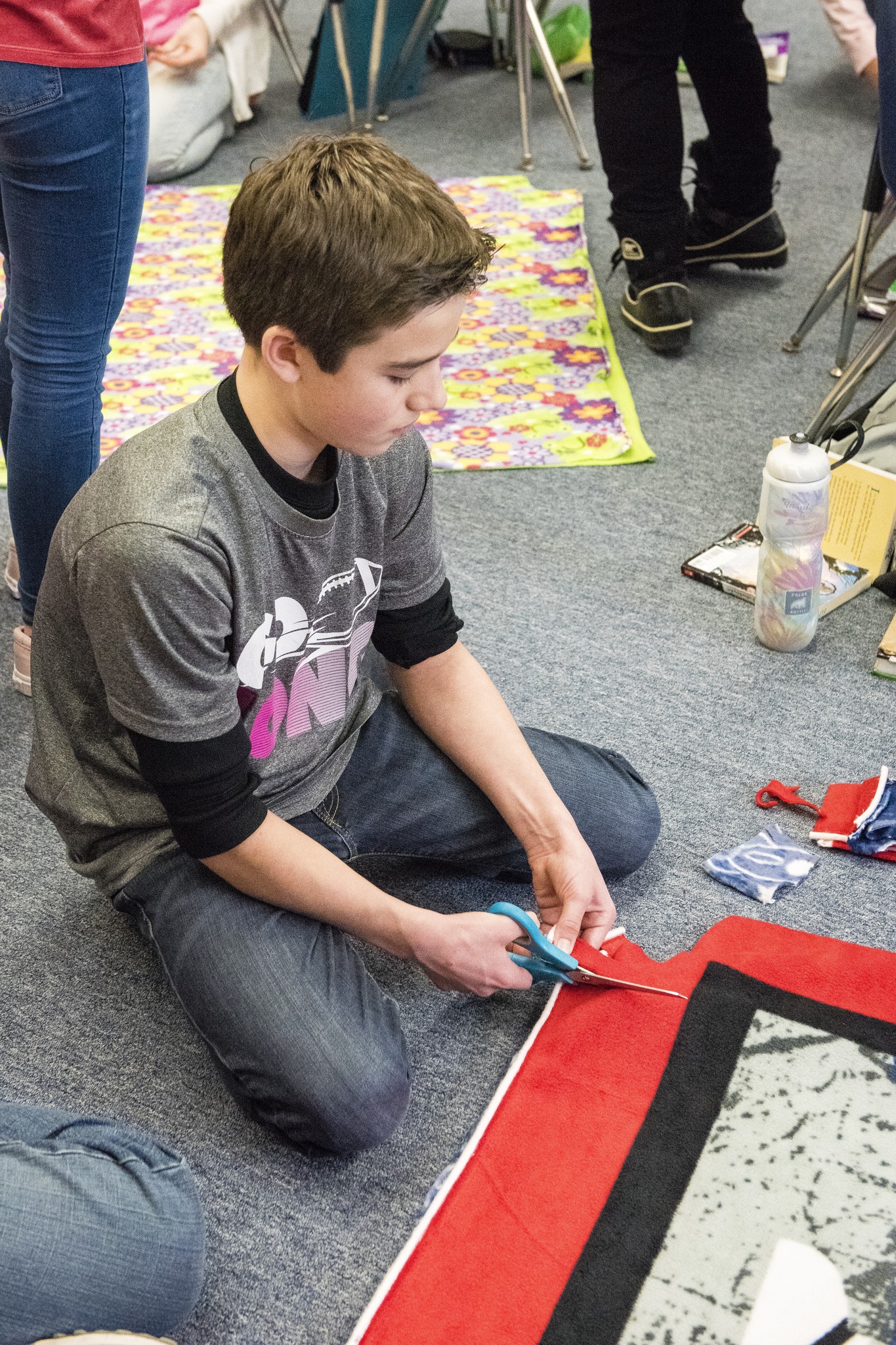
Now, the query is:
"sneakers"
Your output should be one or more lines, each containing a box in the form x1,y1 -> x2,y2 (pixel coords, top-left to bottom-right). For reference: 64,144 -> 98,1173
4,537 -> 19,599
11,626 -> 31,698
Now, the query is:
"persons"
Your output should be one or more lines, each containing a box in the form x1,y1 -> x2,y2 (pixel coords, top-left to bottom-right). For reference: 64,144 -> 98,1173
823,1 -> 881,85
138,1 -> 272,184
0,1104 -> 213,1345
584,1 -> 791,360
19,132 -> 658,1160
0,0 -> 155,692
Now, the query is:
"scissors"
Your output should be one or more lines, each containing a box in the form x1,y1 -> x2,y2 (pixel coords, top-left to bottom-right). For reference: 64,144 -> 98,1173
484,902 -> 689,1002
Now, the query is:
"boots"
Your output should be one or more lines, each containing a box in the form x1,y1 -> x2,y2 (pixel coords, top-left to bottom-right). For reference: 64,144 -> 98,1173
606,200 -> 693,351
686,140 -> 788,271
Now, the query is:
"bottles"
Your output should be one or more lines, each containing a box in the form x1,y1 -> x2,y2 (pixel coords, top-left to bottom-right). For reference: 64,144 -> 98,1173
756,427 -> 833,651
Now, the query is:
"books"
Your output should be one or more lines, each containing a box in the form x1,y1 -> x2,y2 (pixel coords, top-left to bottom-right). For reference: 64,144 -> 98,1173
681,520 -> 875,630
871,613 -> 896,680
773,435 -> 895,571
754,31 -> 790,87
